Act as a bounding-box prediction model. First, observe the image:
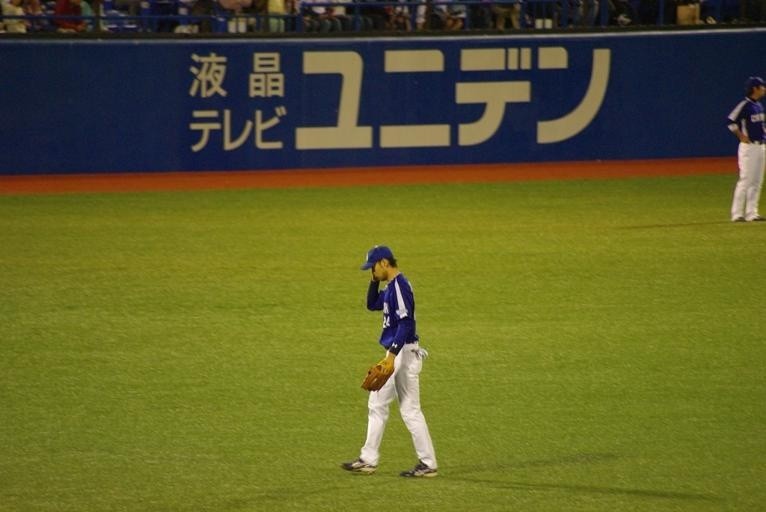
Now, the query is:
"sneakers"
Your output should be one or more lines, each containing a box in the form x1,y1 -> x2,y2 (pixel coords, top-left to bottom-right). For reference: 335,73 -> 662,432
400,458 -> 437,477
735,217 -> 766,221
340,458 -> 378,474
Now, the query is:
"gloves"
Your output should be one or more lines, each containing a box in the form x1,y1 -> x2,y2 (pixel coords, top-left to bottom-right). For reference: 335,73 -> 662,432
413,348 -> 428,360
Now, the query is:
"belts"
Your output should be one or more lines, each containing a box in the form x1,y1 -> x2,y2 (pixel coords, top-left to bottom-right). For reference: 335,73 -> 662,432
384,340 -> 416,349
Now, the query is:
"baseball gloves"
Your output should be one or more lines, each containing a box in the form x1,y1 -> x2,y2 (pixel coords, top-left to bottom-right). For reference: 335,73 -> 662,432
361,359 -> 394,393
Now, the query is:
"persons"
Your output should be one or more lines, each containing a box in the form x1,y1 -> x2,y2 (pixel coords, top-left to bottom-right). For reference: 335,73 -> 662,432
723,76 -> 766,223
0,0 -> 765,44
339,245 -> 438,478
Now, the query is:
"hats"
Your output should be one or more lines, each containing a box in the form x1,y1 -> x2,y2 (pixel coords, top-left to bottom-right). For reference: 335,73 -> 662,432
747,77 -> 765,87
360,245 -> 392,270
